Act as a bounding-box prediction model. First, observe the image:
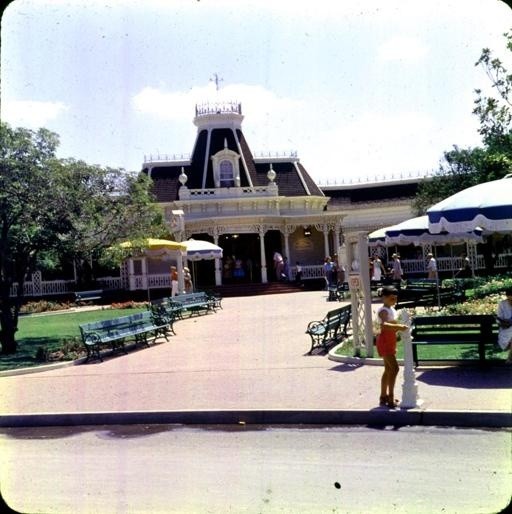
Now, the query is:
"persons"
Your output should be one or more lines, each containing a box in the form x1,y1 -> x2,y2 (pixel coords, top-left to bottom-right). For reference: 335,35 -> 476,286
183,267 -> 192,293
273,251 -> 304,287
223,255 -> 252,282
459,252 -> 472,278
324,256 -> 338,301
370,253 -> 386,281
170,266 -> 178,297
425,253 -> 437,279
375,285 -> 409,408
417,251 -> 423,259
386,253 -> 403,280
496,286 -> 512,368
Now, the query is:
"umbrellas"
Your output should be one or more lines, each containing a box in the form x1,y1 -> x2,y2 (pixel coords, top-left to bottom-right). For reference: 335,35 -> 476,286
427,174 -> 512,234
106,237 -> 187,306
167,238 -> 223,292
368,225 -> 397,265
385,215 -> 484,311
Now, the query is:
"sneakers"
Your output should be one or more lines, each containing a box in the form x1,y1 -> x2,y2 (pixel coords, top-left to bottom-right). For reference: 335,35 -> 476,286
380,396 -> 396,405
380,396 -> 398,410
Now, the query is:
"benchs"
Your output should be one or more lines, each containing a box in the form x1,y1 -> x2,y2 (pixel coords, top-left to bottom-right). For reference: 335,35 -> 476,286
147,304 -> 177,340
169,290 -> 217,319
306,278 -> 511,370
159,296 -> 181,321
78,311 -> 169,365
200,291 -> 224,313
